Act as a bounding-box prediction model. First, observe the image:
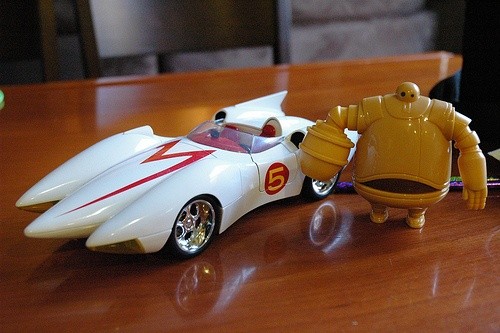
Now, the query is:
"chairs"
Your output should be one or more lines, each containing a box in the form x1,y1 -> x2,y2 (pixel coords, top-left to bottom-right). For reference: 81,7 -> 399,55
78,0 -> 291,79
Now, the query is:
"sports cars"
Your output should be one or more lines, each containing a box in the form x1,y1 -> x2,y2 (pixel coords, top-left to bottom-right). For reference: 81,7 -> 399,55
15,90 -> 359,259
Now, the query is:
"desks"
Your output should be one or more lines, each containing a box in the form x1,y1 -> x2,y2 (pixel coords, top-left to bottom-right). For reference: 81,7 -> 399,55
0,52 -> 500,333
